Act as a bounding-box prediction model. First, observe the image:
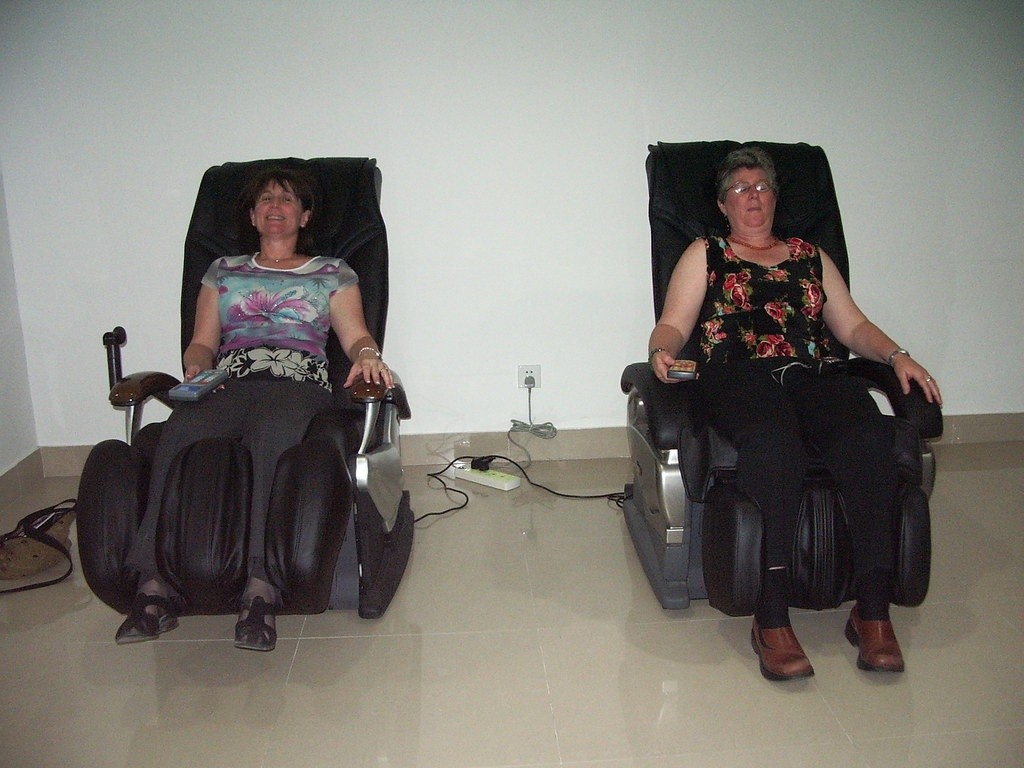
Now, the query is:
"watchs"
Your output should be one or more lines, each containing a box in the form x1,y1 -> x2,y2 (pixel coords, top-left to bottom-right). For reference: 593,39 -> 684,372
889,349 -> 911,369
358,346 -> 382,360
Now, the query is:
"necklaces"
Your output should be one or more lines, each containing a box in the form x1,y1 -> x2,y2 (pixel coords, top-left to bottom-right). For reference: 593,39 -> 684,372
725,233 -> 780,252
266,256 -> 285,263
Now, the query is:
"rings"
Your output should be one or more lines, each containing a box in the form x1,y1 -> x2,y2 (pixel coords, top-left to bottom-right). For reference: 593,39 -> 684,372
380,366 -> 388,376
924,376 -> 932,382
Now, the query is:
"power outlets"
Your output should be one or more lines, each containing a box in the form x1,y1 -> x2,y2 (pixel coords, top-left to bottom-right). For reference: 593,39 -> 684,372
518,364 -> 541,387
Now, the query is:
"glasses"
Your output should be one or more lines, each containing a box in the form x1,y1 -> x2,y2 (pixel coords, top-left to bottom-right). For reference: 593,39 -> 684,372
725,180 -> 774,196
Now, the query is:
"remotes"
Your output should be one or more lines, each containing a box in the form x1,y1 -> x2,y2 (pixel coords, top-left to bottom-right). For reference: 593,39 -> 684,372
170,369 -> 228,402
667,359 -> 699,380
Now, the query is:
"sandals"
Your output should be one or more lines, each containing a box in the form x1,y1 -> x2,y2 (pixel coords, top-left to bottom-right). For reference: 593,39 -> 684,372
114,587 -> 187,645
234,595 -> 277,652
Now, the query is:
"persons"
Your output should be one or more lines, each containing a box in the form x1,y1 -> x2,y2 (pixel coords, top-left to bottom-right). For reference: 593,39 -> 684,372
116,170 -> 394,651
648,148 -> 943,682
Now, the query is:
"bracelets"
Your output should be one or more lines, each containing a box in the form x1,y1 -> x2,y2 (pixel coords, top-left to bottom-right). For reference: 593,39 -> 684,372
649,348 -> 666,372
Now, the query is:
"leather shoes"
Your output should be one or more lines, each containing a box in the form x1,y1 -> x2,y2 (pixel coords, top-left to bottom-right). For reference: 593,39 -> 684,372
845,604 -> 904,672
751,611 -> 815,681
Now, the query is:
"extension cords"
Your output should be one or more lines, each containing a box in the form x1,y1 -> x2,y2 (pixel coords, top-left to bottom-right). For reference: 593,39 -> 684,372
454,465 -> 521,491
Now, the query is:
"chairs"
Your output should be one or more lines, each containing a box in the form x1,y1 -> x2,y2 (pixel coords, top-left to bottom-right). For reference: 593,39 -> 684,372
77,156 -> 415,621
620,140 -> 944,611
